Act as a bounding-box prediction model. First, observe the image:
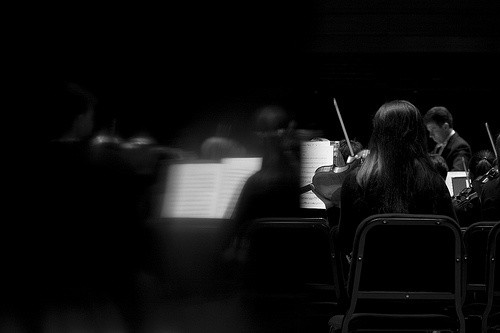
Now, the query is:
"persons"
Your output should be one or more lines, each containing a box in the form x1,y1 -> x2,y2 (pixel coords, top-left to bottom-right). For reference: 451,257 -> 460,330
27,80 -> 160,234
216,104 -> 300,184
309,100 -> 500,333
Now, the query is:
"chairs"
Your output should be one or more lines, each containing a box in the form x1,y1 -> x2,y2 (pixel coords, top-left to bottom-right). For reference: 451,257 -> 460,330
249,213 -> 500,333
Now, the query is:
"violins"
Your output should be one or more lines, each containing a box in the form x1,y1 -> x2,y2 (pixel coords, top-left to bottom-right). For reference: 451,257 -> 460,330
301,149 -> 370,206
